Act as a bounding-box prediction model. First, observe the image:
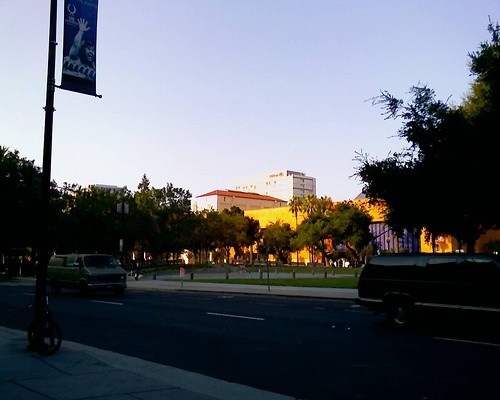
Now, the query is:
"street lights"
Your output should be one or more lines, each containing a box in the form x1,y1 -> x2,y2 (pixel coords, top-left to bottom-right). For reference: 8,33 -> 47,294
261,235 -> 271,291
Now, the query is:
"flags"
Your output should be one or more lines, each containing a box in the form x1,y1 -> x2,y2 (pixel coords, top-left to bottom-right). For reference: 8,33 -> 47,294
60,0 -> 100,98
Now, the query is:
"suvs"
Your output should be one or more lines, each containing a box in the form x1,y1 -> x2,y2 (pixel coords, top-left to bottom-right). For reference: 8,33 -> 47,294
354,249 -> 500,334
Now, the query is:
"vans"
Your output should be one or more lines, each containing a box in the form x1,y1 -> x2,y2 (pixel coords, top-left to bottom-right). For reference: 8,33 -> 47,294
46,253 -> 127,298
